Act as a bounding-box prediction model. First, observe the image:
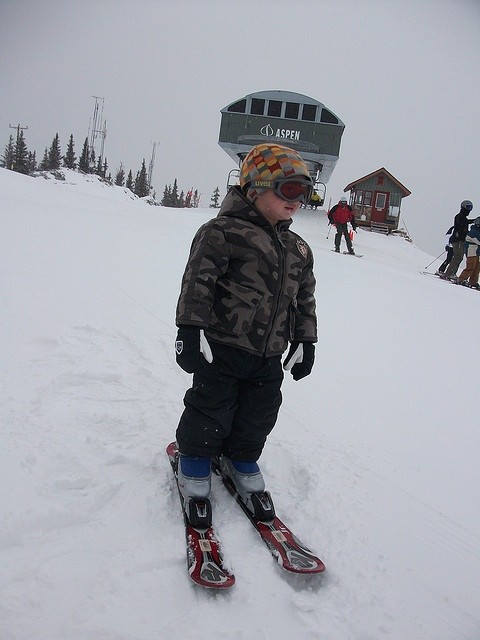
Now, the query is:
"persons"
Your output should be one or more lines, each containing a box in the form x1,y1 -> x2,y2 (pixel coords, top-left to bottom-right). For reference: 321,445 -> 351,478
173,142 -> 320,529
457,216 -> 480,290
327,193 -> 359,255
437,198 -> 471,280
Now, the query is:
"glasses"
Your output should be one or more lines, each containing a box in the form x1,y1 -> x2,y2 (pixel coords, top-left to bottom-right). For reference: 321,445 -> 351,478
242,178 -> 314,207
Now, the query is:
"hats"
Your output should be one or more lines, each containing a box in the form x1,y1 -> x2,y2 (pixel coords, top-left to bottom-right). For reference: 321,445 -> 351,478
340,197 -> 347,204
240,143 -> 313,191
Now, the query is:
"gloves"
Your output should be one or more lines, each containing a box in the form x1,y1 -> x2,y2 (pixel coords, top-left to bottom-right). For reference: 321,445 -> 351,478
174,326 -> 214,375
330,219 -> 335,224
282,340 -> 316,382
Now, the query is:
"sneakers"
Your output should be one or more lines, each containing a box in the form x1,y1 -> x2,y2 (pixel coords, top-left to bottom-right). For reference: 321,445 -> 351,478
468,283 -> 480,290
224,454 -> 265,499
177,452 -> 211,503
457,280 -> 469,287
349,249 -> 354,255
335,247 -> 340,253
445,273 -> 459,280
435,270 -> 444,276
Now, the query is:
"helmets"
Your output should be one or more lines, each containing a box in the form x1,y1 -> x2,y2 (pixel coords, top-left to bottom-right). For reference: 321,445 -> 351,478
461,200 -> 473,211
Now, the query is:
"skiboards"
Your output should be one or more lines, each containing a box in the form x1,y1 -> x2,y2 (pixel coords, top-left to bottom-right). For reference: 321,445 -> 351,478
331,250 -> 363,257
167,440 -> 326,588
434,269 -> 480,290
